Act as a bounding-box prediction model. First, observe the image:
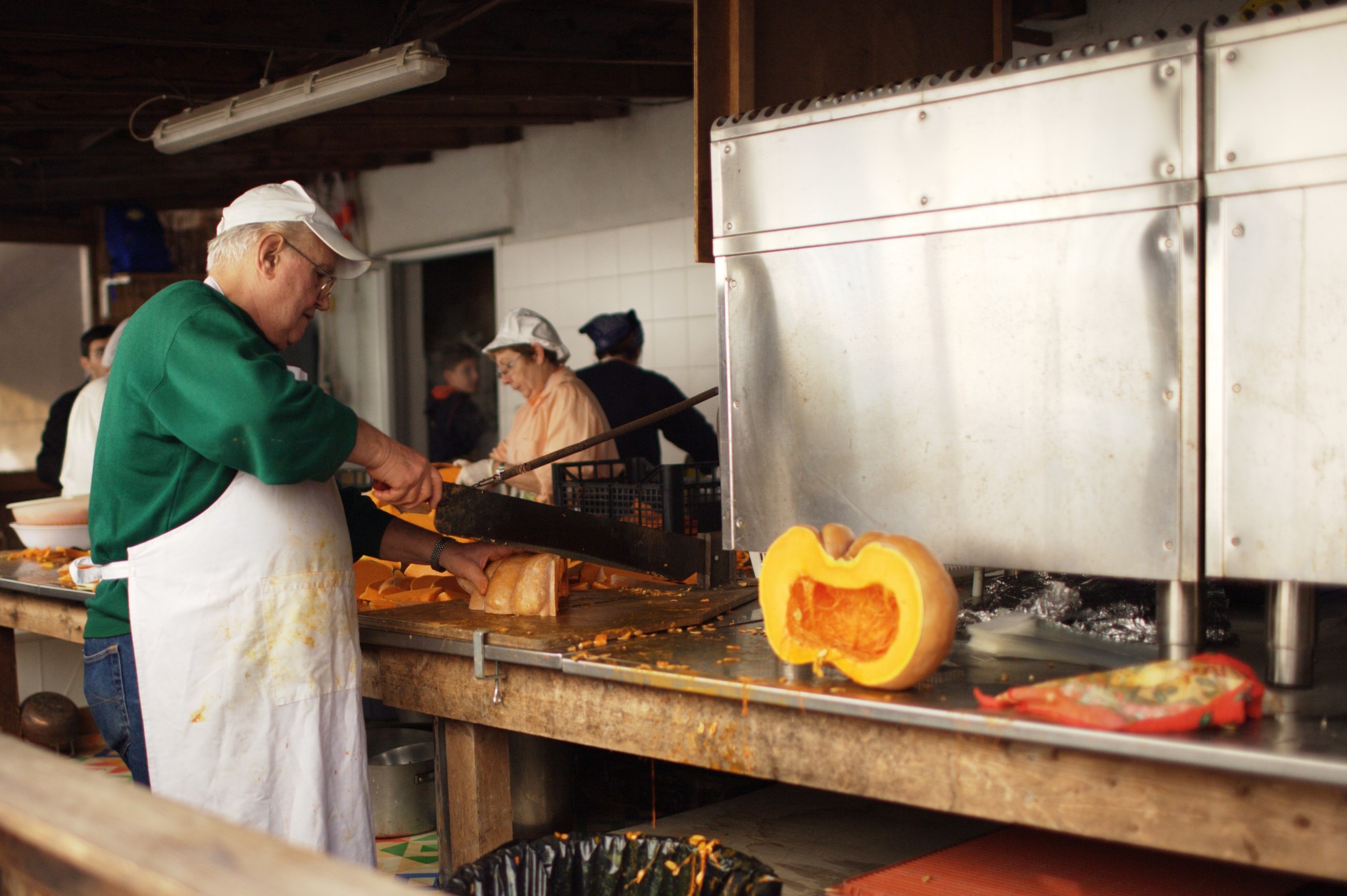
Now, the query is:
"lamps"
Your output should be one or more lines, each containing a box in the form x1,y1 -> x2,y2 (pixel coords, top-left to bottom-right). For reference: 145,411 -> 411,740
151,40 -> 450,154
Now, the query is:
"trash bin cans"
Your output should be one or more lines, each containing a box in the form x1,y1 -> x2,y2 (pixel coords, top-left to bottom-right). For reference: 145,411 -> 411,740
439,827 -> 777,896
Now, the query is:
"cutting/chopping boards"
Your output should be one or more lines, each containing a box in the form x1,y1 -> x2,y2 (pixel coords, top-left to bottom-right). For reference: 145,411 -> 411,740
354,584 -> 759,652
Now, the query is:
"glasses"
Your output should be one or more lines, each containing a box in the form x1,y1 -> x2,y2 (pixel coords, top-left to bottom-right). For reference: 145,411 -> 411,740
496,348 -> 528,377
284,241 -> 339,303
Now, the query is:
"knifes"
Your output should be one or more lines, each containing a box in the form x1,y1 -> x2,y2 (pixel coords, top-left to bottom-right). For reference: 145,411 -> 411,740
370,476 -> 711,582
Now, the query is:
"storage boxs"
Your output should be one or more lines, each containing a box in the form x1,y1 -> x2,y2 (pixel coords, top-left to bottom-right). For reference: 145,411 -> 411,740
553,461 -> 718,536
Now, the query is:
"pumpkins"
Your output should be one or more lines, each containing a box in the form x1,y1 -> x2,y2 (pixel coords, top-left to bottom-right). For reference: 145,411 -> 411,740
15,546 -> 100,589
756,523 -> 957,689
353,464 -> 750,616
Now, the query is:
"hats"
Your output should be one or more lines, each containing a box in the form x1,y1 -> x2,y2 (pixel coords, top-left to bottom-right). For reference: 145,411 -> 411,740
216,179 -> 374,280
580,309 -> 643,352
482,306 -> 570,366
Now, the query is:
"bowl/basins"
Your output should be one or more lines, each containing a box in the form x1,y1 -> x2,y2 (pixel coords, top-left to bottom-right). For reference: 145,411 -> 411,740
6,495 -> 89,525
9,522 -> 90,552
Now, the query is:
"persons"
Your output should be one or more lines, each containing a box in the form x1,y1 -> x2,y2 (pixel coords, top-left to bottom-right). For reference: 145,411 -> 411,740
453,306 -> 624,509
573,308 -> 720,477
70,179 -> 529,871
425,341 -> 486,465
33,320 -> 130,497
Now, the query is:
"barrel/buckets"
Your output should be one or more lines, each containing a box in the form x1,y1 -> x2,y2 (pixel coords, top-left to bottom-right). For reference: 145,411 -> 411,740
508,729 -> 574,841
366,725 -> 437,838
